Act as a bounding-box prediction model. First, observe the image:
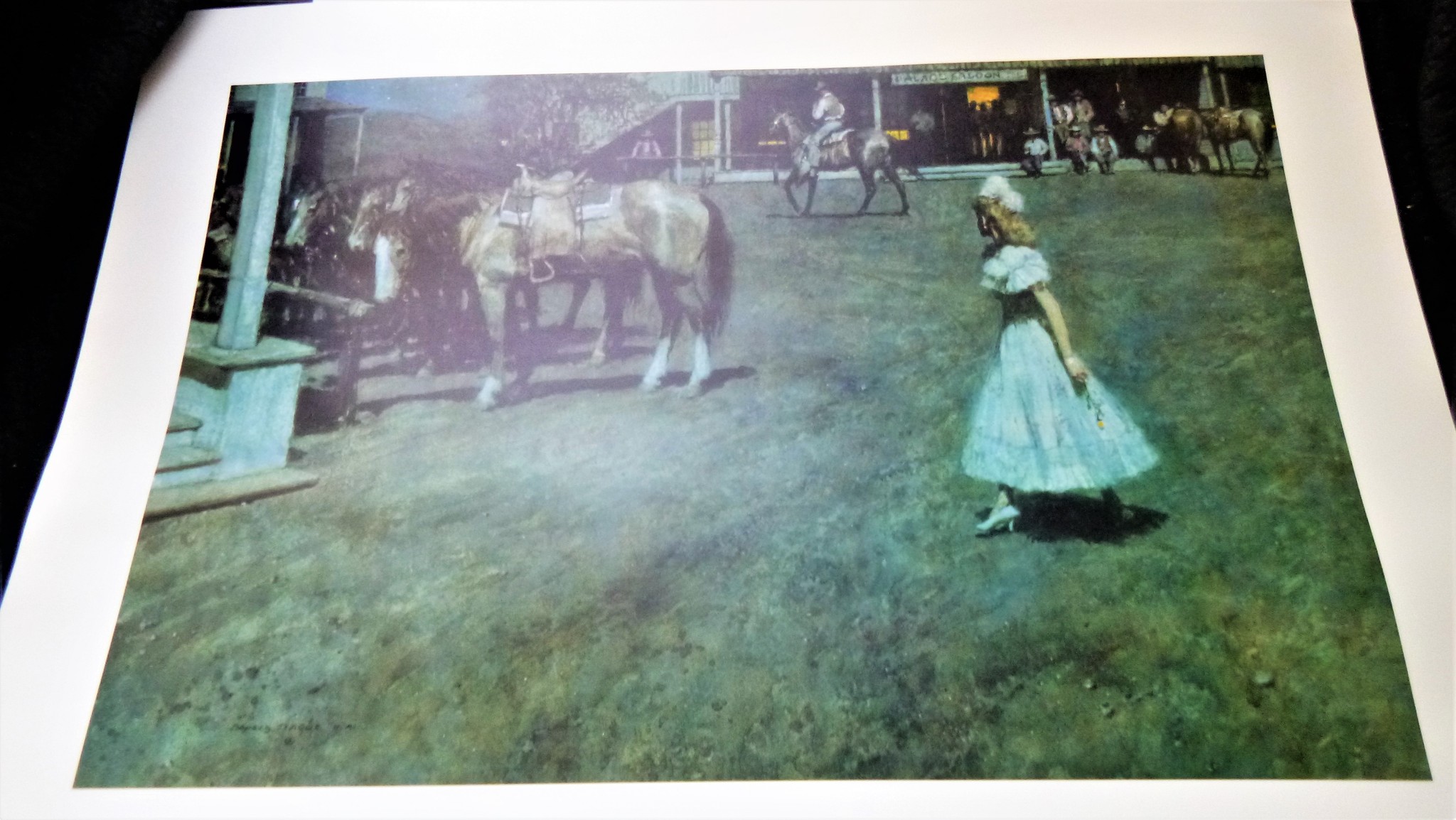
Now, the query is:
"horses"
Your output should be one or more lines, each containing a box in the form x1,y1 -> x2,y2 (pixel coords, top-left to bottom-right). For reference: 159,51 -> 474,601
274,155 -> 736,411
1150,106 -> 1273,178
768,110 -> 910,217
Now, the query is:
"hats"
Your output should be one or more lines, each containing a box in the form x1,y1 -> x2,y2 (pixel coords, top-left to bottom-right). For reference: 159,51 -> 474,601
814,80 -> 826,91
641,131 -> 653,138
1070,126 -> 1081,131
1094,124 -> 1109,132
1142,125 -> 1150,130
1071,90 -> 1083,99
1023,127 -> 1039,136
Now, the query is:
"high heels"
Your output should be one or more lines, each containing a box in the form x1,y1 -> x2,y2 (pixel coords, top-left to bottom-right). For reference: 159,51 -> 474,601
976,506 -> 1020,531
1100,503 -> 1135,530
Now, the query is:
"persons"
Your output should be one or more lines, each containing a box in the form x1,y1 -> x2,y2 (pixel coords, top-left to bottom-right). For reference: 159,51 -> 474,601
803,80 -> 847,174
966,175 -> 1138,535
908,80 -> 1176,175
629,128 -> 664,180
